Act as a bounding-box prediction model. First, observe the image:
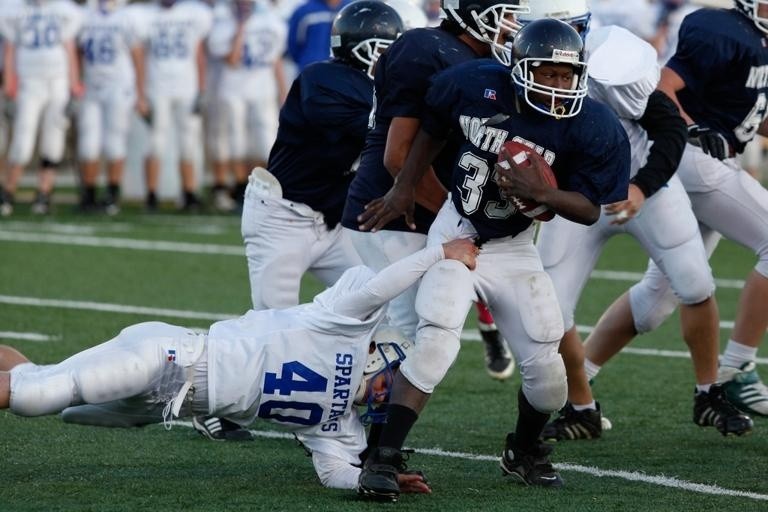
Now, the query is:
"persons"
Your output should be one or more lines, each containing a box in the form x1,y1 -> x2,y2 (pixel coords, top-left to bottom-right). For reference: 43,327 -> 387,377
77,0 -> 149,217
241,0 -> 405,310
515,1 -> 754,443
137,1 -> 213,213
582,0 -> 768,417
402,1 -> 448,29
288,0 -> 352,71
340,0 -> 531,345
0,239 -> 480,494
358,17 -> 631,501
209,1 -> 263,212
242,1 -> 287,170
1,0 -> 84,217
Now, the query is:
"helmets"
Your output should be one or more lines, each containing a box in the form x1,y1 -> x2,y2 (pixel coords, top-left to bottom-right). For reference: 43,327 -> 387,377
330,0 -> 404,82
511,14 -> 591,120
438,1 -> 531,54
517,0 -> 592,56
351,340 -> 410,409
734,1 -> 767,39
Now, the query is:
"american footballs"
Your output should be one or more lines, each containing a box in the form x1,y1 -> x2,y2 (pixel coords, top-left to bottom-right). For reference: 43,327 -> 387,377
495,141 -> 558,222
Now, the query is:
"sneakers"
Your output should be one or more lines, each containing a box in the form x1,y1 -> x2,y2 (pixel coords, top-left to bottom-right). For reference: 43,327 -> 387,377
692,387 -> 754,435
212,190 -> 238,216
190,413 -> 254,443
0,195 -> 17,218
719,358 -> 767,417
28,190 -> 55,218
357,444 -> 402,503
544,399 -> 602,442
479,322 -> 515,382
181,194 -> 206,218
72,195 -> 99,213
143,193 -> 162,214
104,196 -> 125,219
499,431 -> 565,489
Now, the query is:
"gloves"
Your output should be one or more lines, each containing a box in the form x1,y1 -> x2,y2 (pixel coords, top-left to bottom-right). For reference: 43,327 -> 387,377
687,123 -> 732,161
